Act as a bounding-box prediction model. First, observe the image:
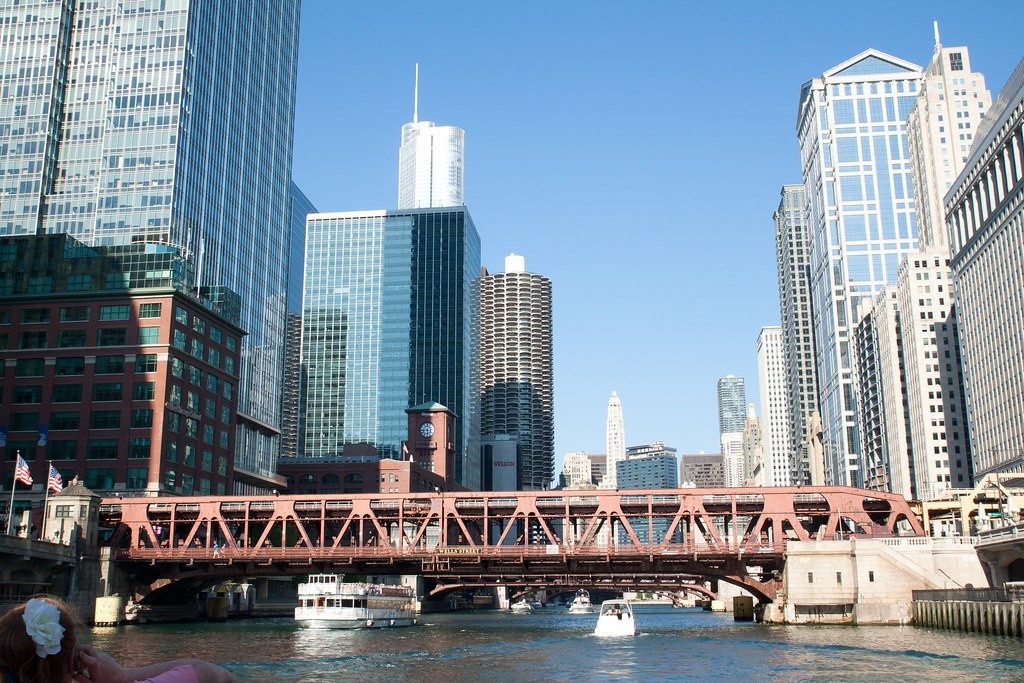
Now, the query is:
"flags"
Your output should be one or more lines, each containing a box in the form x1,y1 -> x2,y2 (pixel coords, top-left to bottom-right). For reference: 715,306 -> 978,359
48,463 -> 62,492
13,453 -> 33,486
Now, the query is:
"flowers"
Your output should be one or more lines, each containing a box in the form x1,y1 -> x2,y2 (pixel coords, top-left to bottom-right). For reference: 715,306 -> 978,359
21,597 -> 66,659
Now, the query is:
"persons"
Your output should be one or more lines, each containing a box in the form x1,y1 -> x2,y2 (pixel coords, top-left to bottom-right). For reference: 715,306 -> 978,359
212,541 -> 220,559
0,595 -> 235,683
623,606 -> 628,612
605,607 -> 613,614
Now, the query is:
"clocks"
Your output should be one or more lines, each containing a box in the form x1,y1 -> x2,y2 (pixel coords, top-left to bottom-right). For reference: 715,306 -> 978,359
419,421 -> 435,438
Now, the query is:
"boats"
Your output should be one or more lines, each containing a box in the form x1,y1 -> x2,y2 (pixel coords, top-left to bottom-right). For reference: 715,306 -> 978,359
594,598 -> 635,636
511,597 -> 535,615
294,572 -> 422,629
531,600 -> 542,609
123,596 -> 199,624
569,594 -> 594,614
